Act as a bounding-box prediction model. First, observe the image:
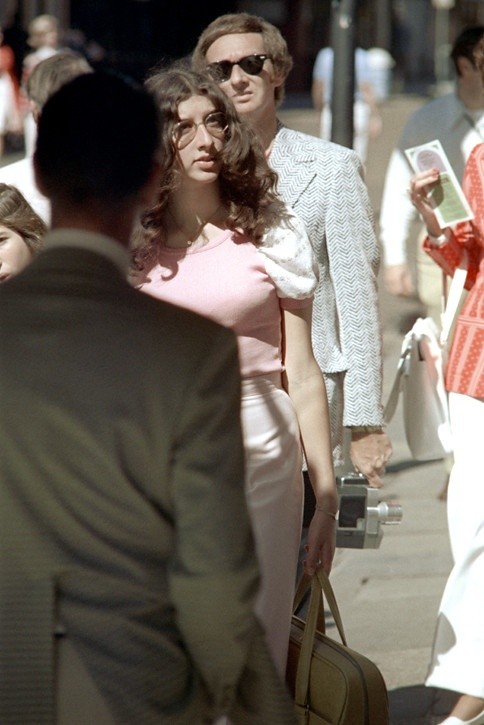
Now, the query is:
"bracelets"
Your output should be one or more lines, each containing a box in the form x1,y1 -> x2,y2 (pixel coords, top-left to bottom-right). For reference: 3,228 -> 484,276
350,426 -> 383,433
314,504 -> 340,521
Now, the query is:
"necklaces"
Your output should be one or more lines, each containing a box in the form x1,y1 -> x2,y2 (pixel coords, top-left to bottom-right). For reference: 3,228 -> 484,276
173,205 -> 222,245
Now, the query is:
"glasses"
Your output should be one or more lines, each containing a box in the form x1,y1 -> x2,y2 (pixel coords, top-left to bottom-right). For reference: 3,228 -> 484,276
205,53 -> 273,83
171,112 -> 230,141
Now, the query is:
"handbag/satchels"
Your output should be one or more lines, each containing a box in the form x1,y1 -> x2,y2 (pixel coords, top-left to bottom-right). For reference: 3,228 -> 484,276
384,316 -> 452,462
281,563 -> 390,725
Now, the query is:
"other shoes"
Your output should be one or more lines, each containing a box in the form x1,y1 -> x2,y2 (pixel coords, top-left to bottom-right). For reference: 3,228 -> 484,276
434,707 -> 484,725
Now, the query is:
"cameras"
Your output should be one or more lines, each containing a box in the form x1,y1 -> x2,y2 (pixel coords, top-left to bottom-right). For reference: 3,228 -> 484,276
334,472 -> 404,550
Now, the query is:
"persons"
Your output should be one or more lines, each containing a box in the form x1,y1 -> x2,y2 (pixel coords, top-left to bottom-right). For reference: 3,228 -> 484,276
191,14 -> 392,634
0,72 -> 300,725
312,24 -> 397,164
379,24 -> 484,346
0,15 -> 92,283
128,61 -> 340,682
410,144 -> 484,725
